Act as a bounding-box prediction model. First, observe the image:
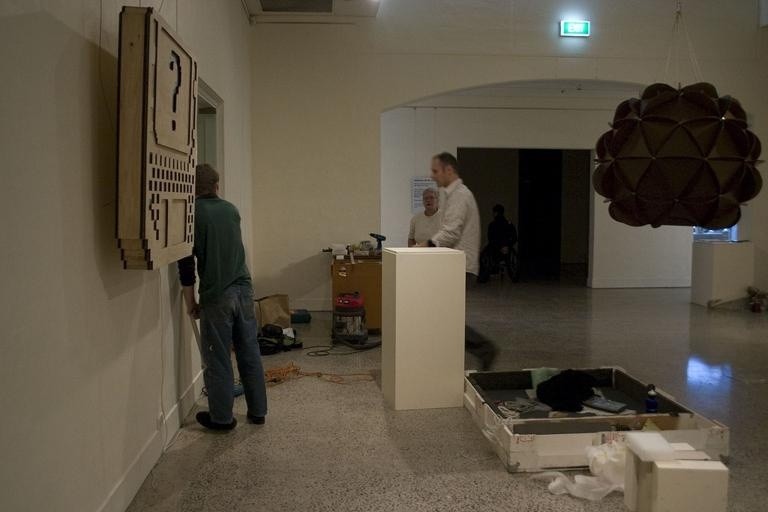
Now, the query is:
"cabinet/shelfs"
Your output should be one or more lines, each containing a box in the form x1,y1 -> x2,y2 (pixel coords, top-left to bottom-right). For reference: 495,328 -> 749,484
330,253 -> 382,329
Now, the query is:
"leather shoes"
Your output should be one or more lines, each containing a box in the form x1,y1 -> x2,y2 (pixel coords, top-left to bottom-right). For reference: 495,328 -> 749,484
247,411 -> 265,424
196,411 -> 237,430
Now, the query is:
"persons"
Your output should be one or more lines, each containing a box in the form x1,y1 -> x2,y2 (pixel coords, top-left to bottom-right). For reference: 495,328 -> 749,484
178,164 -> 268,431
477,204 -> 517,283
407,188 -> 443,247
415,152 -> 504,371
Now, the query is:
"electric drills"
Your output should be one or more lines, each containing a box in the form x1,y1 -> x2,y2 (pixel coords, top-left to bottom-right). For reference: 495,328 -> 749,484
368,232 -> 386,250
